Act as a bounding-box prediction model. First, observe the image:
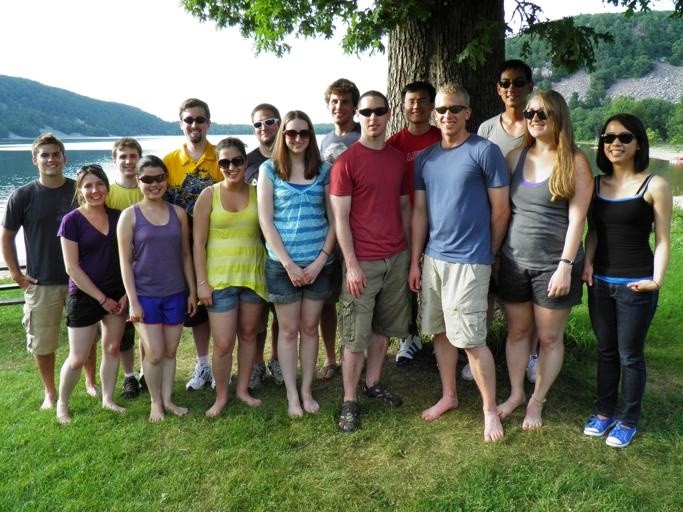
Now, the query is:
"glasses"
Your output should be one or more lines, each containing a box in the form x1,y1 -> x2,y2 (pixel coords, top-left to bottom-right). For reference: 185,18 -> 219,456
601,132 -> 634,145
139,172 -> 169,184
523,109 -> 552,121
252,118 -> 279,128
284,129 -> 311,139
500,78 -> 528,88
218,155 -> 245,169
359,106 -> 389,117
434,104 -> 467,114
182,115 -> 207,125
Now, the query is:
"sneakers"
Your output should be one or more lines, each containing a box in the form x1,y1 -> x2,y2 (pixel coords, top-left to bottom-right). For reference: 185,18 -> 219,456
362,381 -> 402,407
186,363 -> 234,391
462,364 -> 474,381
527,354 -> 539,383
395,334 -> 423,365
121,376 -> 148,398
605,421 -> 636,449
248,361 -> 266,390
584,415 -> 617,437
338,400 -> 359,432
268,359 -> 284,385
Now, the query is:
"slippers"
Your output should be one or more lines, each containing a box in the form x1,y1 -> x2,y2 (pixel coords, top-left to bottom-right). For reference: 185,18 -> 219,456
317,363 -> 338,380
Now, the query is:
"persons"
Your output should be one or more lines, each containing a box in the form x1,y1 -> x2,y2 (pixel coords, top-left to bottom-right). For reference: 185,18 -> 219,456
54,163 -> 124,422
582,113 -> 673,446
0,133 -> 99,411
101,57 -> 593,444
